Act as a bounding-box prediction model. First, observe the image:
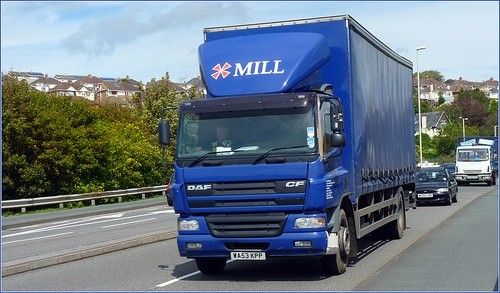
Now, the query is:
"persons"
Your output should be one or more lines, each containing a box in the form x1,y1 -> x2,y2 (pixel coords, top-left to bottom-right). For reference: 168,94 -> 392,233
205,123 -> 241,150
473,151 -> 481,159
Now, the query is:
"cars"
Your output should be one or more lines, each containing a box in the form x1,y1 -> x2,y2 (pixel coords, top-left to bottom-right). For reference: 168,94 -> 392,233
441,163 -> 456,178
415,167 -> 459,206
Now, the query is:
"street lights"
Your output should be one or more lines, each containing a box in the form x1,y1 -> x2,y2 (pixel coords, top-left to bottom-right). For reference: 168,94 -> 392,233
416,46 -> 427,167
459,117 -> 468,142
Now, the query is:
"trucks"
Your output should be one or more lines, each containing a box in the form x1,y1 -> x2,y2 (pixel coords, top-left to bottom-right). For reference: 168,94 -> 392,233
157,14 -> 416,276
455,135 -> 499,186
